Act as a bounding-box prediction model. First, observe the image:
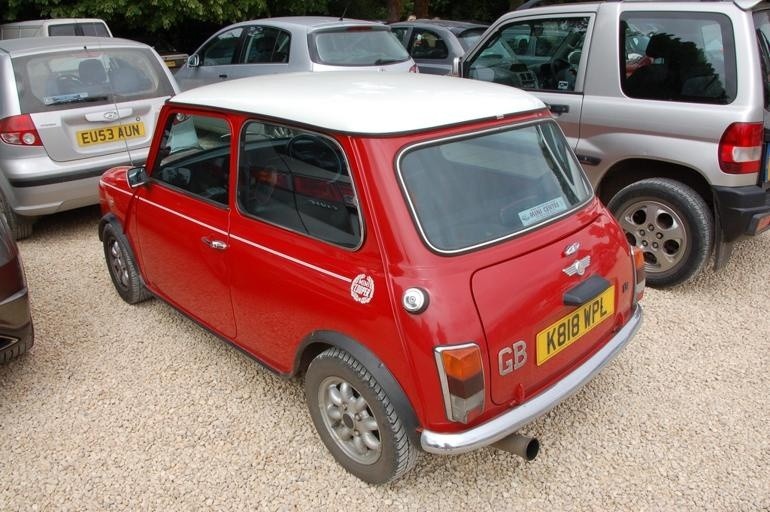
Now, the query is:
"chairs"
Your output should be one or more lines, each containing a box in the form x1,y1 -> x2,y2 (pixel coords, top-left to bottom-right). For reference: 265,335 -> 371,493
76,59 -> 111,97
624,32 -> 673,100
